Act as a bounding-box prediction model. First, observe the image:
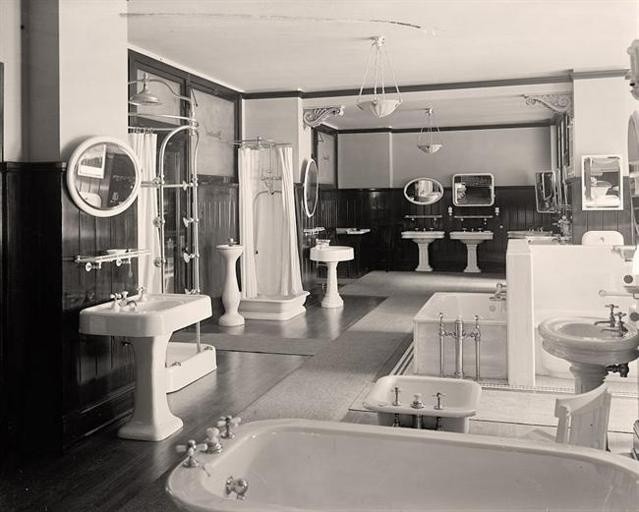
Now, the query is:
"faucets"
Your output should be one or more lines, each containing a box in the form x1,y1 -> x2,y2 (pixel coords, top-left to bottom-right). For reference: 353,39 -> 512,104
119,289 -> 138,309
472,228 -> 474,232
600,312 -> 627,335
423,228 -> 426,231
594,305 -> 617,326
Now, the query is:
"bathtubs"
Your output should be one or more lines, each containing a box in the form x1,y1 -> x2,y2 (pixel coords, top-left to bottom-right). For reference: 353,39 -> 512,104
166,418 -> 639,512
412,293 -> 507,376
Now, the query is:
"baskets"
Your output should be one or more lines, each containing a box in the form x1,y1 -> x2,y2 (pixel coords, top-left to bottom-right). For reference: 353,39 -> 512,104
416,144 -> 442,154
357,99 -> 402,119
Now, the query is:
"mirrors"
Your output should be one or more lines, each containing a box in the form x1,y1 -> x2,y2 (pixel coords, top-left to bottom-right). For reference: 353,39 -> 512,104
398,170 -> 495,208
303,156 -> 320,217
535,153 -> 623,214
65,134 -> 143,217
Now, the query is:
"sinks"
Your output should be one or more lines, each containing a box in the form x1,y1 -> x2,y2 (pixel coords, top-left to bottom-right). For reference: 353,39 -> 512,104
364,375 -> 482,433
450,231 -> 493,242
401,231 -> 446,239
507,230 -> 553,239
79,294 -> 212,336
538,315 -> 639,365
309,246 -> 354,263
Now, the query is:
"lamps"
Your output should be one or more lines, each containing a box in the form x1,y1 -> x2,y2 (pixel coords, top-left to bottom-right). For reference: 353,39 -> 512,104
355,33 -> 444,155
